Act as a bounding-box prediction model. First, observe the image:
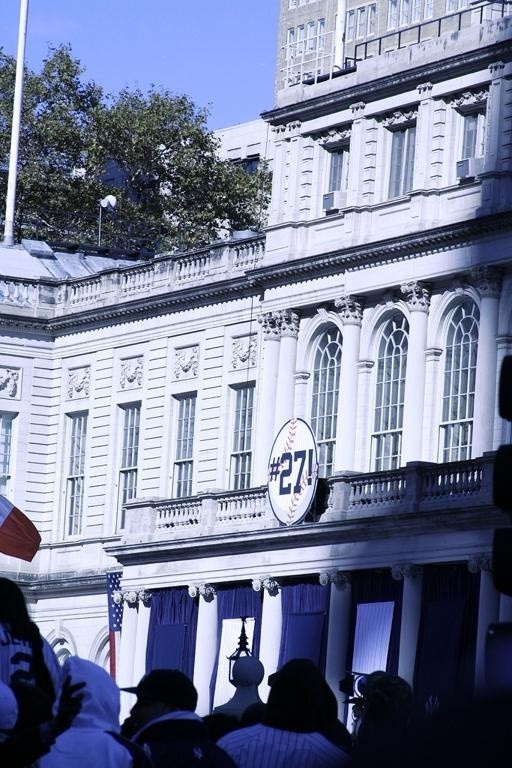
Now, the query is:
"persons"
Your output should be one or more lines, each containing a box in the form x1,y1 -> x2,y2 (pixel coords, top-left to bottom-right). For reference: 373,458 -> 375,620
0,652 -> 512,768
0,576 -> 63,712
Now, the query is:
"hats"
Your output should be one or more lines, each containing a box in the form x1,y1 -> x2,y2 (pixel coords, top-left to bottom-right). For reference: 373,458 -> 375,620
119,670 -> 197,699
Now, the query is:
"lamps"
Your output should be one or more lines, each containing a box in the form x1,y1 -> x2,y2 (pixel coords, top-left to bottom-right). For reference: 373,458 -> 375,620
114,587 -> 152,604
318,569 -> 351,588
187,581 -> 218,602
389,562 -> 416,581
467,553 -> 492,575
251,574 -> 279,593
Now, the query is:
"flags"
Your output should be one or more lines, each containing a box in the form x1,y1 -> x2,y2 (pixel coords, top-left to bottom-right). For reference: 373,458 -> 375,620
1,491 -> 43,564
